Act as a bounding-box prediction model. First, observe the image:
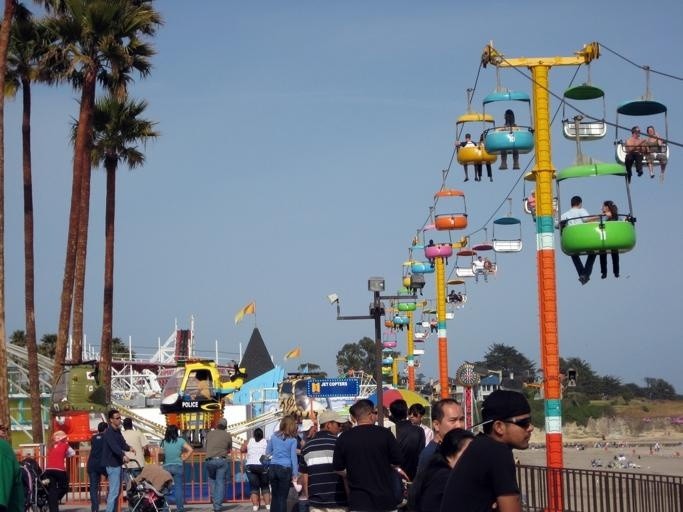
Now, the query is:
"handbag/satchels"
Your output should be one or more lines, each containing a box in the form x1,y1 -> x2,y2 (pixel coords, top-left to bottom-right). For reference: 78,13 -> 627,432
259,455 -> 270,465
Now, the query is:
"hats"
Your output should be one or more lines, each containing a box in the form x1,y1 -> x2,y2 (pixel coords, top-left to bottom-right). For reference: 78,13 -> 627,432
52,430 -> 67,443
467,388 -> 530,429
318,409 -> 347,423
218,418 -> 227,429
300,419 -> 314,432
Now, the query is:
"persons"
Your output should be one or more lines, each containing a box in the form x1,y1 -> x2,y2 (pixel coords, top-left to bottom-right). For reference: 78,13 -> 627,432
645,126 -> 666,181
240,427 -> 271,511
85,422 -> 117,511
120,418 -> 148,486
589,201 -> 620,280
553,195 -> 596,284
99,409 -> 136,512
439,385 -> 532,511
203,417 -> 232,512
260,396 -> 472,511
188,370 -> 211,402
379,101 -> 535,335
0,419 -> 25,511
158,424 -> 192,511
624,126 -> 643,183
529,439 -> 682,470
45,430 -> 75,511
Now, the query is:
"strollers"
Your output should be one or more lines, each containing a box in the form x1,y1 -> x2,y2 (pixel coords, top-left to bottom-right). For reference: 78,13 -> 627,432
17,457 -> 50,512
120,459 -> 171,512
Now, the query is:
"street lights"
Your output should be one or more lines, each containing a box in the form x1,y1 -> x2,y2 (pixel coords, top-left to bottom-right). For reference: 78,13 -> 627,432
367,274 -> 426,430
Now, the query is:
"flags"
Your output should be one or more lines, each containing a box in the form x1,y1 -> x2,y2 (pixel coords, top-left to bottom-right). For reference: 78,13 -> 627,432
283,345 -> 299,361
234,301 -> 254,325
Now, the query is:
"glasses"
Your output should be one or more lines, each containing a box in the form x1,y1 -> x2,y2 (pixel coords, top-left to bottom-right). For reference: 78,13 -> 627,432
112,417 -> 119,419
503,416 -> 530,429
407,410 -> 417,417
370,410 -> 377,415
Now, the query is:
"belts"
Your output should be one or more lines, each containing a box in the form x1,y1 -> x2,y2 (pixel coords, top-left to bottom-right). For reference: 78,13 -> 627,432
205,456 -> 225,460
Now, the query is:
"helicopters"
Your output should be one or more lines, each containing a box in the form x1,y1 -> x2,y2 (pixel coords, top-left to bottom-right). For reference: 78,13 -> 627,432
273,370 -> 334,419
157,359 -> 246,415
47,359 -> 110,413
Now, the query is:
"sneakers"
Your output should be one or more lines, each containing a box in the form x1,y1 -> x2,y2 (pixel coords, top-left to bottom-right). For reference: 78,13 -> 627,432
265,504 -> 270,509
252,505 -> 259,511
